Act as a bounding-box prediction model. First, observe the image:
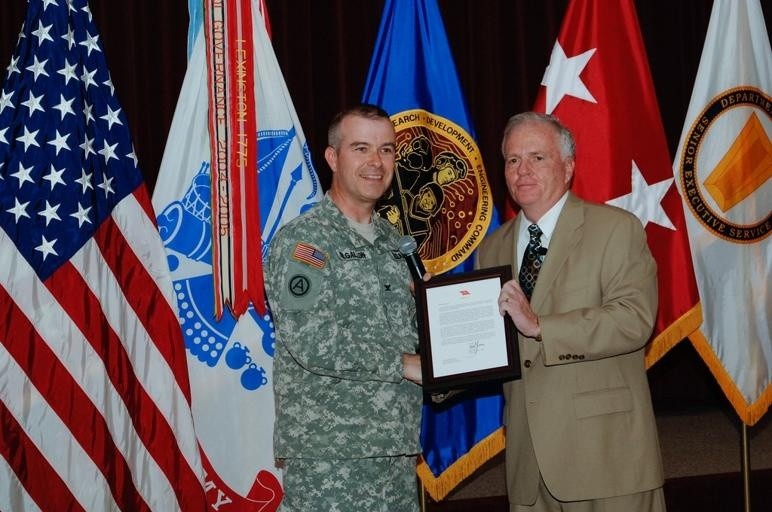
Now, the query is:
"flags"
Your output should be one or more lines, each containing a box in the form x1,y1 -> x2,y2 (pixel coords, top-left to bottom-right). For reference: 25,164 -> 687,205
0,0 -> 772,512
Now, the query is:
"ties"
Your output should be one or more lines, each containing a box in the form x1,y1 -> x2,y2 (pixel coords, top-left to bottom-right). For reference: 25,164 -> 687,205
516,224 -> 545,308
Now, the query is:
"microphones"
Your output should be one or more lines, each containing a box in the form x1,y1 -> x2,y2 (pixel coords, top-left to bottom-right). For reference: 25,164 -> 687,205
398,235 -> 427,283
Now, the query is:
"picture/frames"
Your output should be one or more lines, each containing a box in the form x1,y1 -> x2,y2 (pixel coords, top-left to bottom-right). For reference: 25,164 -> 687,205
417,264 -> 521,395
